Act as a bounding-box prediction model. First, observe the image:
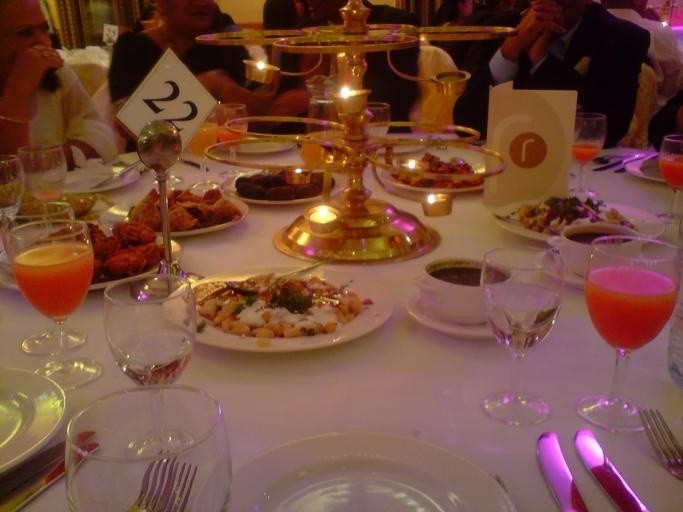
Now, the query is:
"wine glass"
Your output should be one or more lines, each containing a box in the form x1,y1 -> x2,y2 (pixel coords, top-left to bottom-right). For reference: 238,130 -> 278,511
657,134 -> 683,224
0,141 -> 100,388
479,246 -> 566,426
189,100 -> 248,192
100,271 -> 197,458
578,235 -> 680,430
569,112 -> 608,196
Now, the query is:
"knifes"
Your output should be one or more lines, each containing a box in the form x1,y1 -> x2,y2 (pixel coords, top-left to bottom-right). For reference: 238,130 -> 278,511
1,431 -> 99,511
535,428 -> 649,511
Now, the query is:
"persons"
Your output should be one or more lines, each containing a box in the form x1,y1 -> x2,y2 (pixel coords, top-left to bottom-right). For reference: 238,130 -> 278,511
647,89 -> 682,152
109,1 -> 273,147
261,1 -> 421,134
1,1 -> 117,185
434,1 -> 682,148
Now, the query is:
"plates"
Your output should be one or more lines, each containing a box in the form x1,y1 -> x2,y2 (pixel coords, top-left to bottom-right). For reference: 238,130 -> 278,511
533,248 -> 591,286
491,197 -> 666,243
234,167 -> 335,203
128,189 -> 251,238
162,268 -> 395,349
0,367 -> 65,477
363,165 -> 508,197
405,284 -> 558,337
228,432 -> 517,510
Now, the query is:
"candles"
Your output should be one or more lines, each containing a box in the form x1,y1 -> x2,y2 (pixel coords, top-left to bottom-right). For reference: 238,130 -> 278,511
305,203 -> 340,233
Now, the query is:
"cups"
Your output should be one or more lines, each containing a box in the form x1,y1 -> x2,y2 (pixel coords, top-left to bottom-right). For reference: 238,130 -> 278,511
364,103 -> 391,137
306,96 -> 345,143
64,387 -> 232,509
420,257 -> 511,328
547,222 -> 645,279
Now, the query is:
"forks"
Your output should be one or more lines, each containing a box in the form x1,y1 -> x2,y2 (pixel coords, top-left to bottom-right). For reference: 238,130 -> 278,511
134,450 -> 197,511
639,409 -> 682,481
